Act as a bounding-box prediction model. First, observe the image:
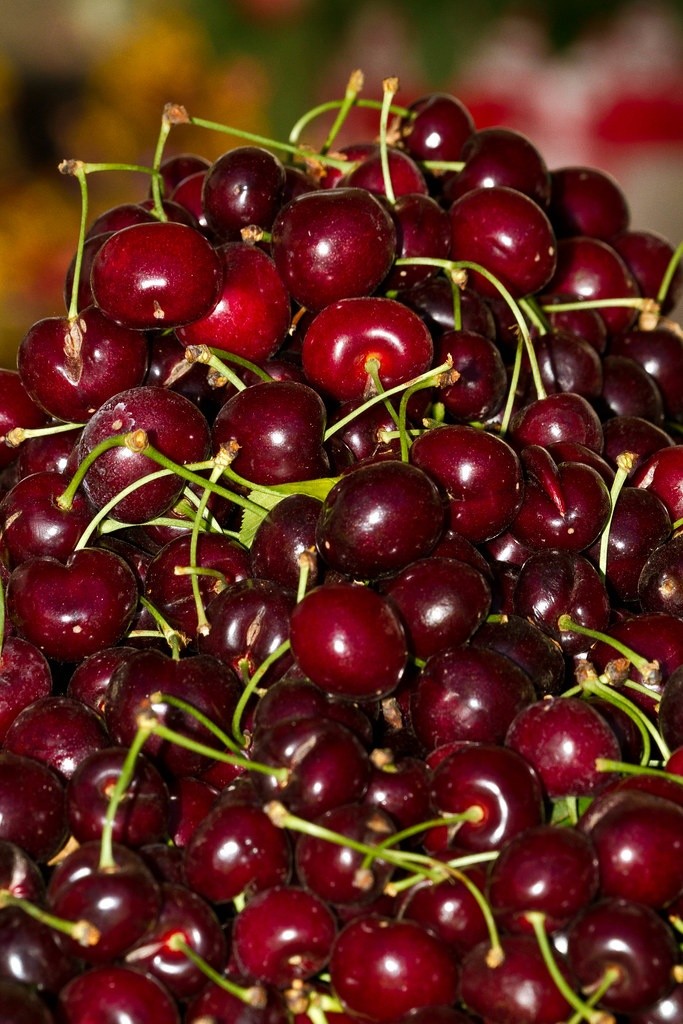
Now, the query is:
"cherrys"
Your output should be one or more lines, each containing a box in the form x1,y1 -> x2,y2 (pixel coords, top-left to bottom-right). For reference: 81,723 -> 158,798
0,68 -> 683,1024
